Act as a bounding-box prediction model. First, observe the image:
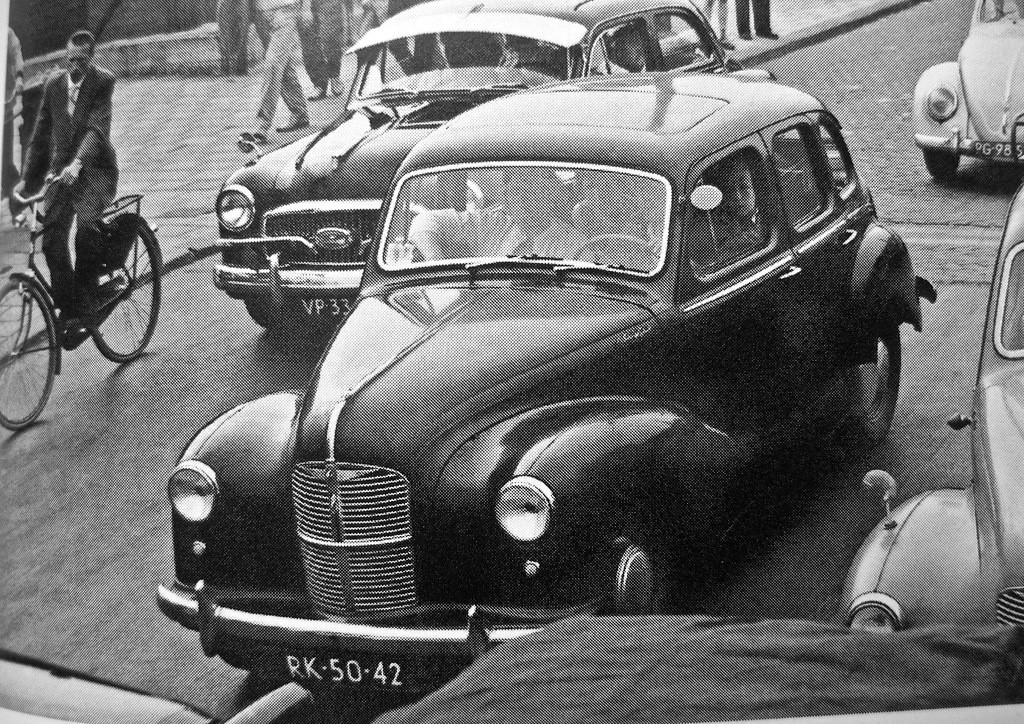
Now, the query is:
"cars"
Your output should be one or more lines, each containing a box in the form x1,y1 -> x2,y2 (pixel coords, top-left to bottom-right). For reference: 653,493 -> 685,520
153,74 -> 937,698
911,0 -> 1024,182
840,181 -> 1024,634
210,0 -> 776,335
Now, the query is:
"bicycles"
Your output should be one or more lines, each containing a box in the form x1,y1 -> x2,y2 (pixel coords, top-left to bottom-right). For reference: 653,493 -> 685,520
0,175 -> 166,431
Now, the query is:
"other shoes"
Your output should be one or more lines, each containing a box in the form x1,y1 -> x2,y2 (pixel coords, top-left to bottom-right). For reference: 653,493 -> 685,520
276,120 -> 309,131
306,87 -> 326,100
254,135 -> 265,144
331,77 -> 344,95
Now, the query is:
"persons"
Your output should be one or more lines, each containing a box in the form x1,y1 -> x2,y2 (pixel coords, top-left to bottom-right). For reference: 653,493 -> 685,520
3,28 -> 26,227
717,158 -> 766,238
405,169 -> 587,281
236,0 -> 310,143
297,0 -> 346,102
13,30 -> 121,327
